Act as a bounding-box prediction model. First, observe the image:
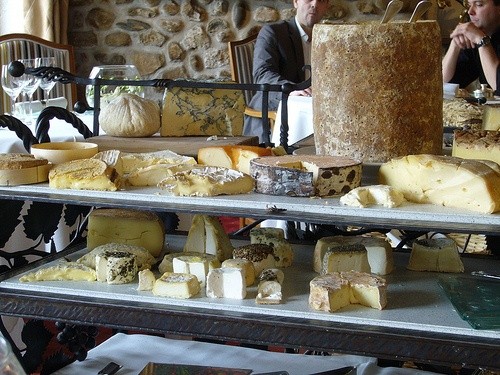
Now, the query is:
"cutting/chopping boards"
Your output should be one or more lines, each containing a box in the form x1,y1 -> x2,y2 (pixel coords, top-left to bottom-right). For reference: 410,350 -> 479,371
88,132 -> 260,157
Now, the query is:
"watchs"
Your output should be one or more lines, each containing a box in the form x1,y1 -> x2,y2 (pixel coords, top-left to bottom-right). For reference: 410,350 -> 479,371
476,35 -> 491,48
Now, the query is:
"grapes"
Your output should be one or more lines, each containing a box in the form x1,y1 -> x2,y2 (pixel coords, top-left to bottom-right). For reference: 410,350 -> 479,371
56,321 -> 99,363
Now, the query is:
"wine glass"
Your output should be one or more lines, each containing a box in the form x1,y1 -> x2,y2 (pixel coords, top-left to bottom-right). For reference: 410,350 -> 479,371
17,59 -> 42,125
0,64 -> 26,131
36,57 -> 58,108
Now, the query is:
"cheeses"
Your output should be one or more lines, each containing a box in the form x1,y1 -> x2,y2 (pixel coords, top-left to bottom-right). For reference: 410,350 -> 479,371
339,89 -> 500,214
19,209 -> 464,312
99,76 -> 245,137
48,146 -> 361,198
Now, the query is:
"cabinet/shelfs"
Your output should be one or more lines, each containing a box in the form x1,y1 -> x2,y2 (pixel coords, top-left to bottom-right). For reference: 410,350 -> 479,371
0,62 -> 500,375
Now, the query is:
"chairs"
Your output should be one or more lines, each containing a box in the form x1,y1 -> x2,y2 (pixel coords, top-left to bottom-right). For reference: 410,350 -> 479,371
228,33 -> 277,129
0,33 -> 76,118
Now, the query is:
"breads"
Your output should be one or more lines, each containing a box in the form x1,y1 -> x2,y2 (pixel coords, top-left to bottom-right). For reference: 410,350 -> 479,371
0,153 -> 48,169
311,17 -> 443,164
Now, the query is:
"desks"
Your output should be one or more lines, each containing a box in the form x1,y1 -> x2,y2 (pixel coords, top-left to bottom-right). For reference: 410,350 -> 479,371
271,94 -> 500,148
0,112 -> 107,268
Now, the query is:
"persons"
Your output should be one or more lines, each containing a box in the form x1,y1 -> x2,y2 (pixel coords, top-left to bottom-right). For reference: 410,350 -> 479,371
442,0 -> 500,96
241,0 -> 329,144
